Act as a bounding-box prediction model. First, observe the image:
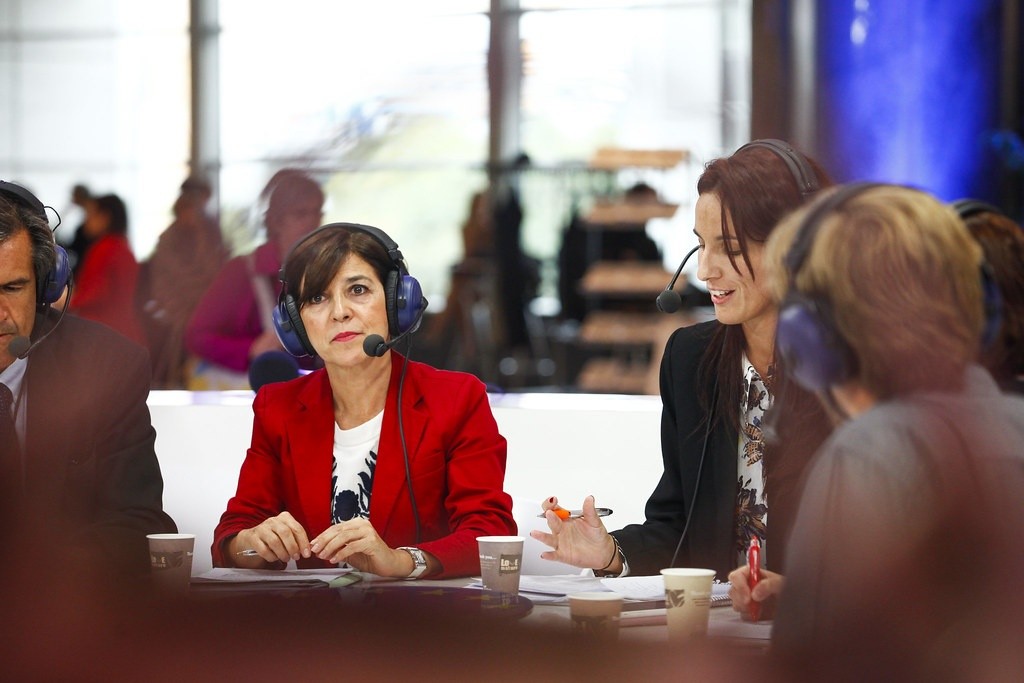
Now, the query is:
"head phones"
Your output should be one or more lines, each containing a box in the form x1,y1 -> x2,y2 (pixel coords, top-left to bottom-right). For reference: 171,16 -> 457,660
0,181 -> 70,305
271,223 -> 428,358
775,180 -> 1000,392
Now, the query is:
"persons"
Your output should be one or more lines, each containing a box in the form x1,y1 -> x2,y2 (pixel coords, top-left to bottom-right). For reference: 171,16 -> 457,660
211,223 -> 518,581
529,138 -> 834,579
52,152 -> 664,391
729,180 -> 1024,683
0,182 -> 178,579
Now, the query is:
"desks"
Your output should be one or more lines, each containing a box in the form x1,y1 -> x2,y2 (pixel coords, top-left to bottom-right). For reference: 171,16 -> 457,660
154,567 -> 776,683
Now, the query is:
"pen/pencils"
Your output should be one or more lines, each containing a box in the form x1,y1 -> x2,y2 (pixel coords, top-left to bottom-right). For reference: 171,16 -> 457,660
536,508 -> 613,520
236,549 -> 257,556
746,535 -> 760,626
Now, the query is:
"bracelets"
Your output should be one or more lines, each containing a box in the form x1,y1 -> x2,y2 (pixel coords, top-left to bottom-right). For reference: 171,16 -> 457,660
592,533 -> 616,571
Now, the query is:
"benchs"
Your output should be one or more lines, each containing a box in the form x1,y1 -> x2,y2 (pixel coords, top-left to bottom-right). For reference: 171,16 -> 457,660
148,387 -> 663,576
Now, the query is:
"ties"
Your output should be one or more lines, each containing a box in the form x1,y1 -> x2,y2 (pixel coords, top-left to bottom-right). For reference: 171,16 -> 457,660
0,384 -> 14,553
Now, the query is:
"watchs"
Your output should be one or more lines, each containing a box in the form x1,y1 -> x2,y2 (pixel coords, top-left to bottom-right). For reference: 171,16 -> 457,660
395,547 -> 428,580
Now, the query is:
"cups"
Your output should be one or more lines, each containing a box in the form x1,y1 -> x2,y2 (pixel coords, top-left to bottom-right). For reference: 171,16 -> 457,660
567,592 -> 622,638
659,567 -> 717,640
147,534 -> 195,590
476,536 -> 523,594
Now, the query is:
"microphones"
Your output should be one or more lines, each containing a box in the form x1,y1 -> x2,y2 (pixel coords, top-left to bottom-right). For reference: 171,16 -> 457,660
9,280 -> 74,359
362,299 -> 427,355
656,246 -> 700,313
760,352 -> 792,448
